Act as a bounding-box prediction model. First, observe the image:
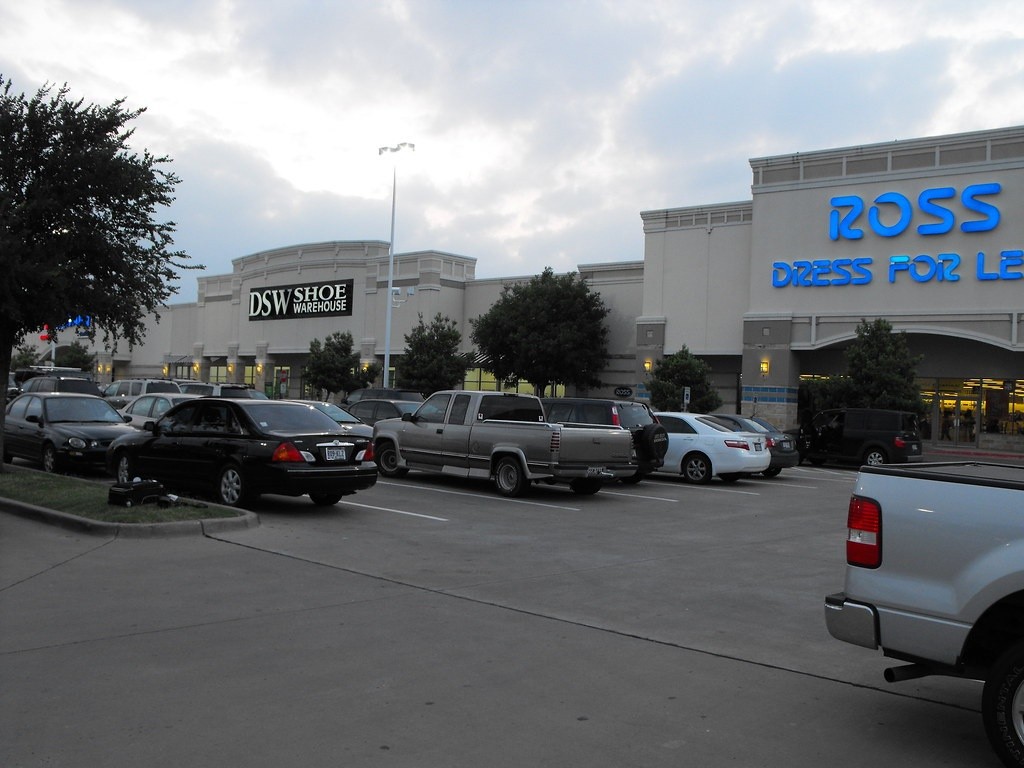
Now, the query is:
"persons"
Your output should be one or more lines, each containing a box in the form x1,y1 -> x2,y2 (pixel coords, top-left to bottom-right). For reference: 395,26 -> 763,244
963,409 -> 976,440
986,416 -> 999,434
939,416 -> 952,440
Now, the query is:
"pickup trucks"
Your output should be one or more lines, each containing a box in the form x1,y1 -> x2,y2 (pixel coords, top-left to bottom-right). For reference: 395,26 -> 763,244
373,390 -> 640,499
823,455 -> 1024,768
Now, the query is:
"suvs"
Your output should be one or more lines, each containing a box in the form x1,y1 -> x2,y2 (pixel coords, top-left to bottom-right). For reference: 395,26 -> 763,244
99,378 -> 184,412
179,381 -> 271,399
11,366 -> 84,389
538,397 -> 669,486
21,375 -> 105,400
337,390 -> 427,409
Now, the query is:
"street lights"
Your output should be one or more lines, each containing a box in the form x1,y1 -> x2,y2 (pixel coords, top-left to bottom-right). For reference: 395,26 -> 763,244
367,140 -> 417,388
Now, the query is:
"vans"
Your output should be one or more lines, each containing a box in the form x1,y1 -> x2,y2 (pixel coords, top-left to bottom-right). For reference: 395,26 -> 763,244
780,408 -> 925,467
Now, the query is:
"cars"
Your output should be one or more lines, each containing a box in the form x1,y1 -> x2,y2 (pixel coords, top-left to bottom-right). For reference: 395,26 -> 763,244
276,399 -> 374,436
708,413 -> 799,479
105,397 -> 379,510
345,399 -> 440,431
4,391 -> 145,474
655,411 -> 772,484
8,374 -> 21,398
114,393 -> 209,432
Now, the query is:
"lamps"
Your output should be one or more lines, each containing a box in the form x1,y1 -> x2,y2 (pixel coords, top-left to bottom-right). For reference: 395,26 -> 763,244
759,361 -> 768,373
643,361 -> 650,372
